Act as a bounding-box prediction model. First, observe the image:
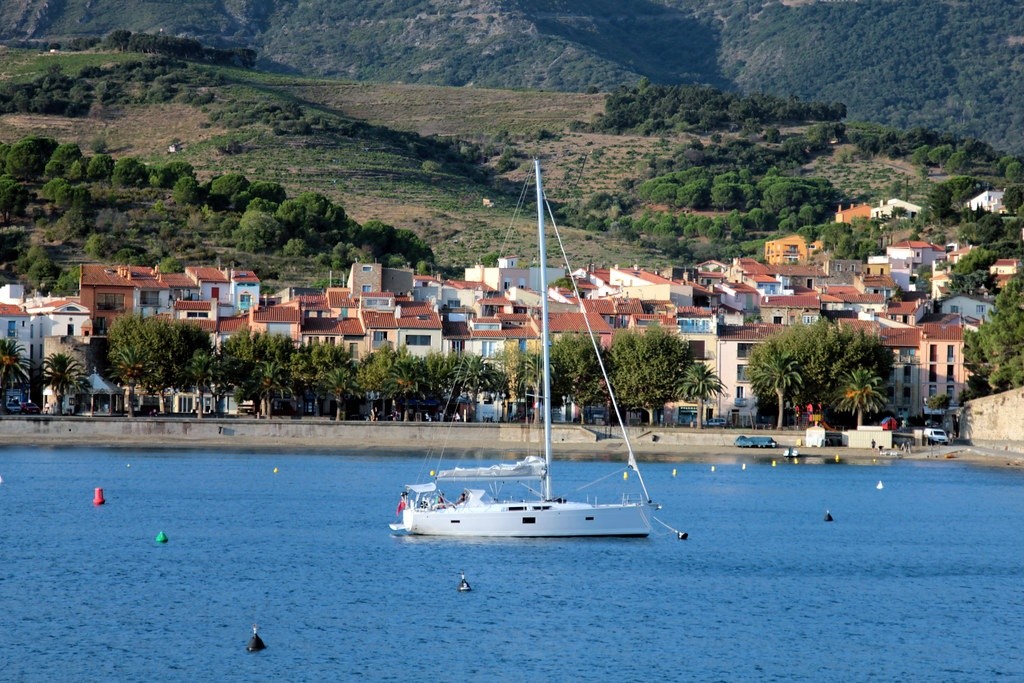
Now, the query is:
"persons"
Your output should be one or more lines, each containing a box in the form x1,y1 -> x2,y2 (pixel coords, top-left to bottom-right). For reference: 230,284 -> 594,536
901,442 -> 911,456
438,493 -> 446,509
871,439 -> 876,453
453,494 -> 465,508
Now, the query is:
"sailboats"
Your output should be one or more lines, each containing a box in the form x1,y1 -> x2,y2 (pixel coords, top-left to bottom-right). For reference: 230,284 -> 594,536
390,159 -> 654,538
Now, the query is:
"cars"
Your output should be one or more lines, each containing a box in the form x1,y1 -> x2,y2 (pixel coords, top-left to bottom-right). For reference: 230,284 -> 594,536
702,418 -> 727,428
6,402 -> 22,414
19,403 -> 40,415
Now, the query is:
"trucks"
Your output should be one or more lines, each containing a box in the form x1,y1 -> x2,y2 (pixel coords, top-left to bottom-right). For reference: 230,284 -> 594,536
924,428 -> 949,445
